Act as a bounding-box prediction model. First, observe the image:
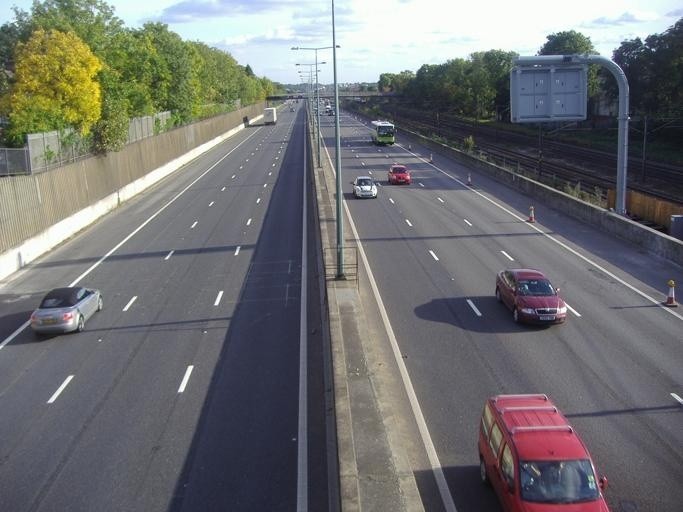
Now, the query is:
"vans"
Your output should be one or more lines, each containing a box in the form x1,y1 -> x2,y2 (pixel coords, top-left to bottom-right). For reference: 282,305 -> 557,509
473,388 -> 610,512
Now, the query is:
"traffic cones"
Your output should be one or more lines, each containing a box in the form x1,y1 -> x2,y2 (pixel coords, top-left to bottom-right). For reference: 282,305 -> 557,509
341,109 -> 368,125
463,172 -> 475,186
408,144 -> 413,151
524,205 -> 537,224
660,276 -> 678,307
428,151 -> 433,163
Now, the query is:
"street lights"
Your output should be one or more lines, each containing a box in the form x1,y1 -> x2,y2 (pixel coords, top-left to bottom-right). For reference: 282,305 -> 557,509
287,43 -> 342,167
295,61 -> 330,138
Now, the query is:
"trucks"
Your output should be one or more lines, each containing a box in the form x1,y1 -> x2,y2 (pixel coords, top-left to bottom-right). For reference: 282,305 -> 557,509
262,106 -> 278,125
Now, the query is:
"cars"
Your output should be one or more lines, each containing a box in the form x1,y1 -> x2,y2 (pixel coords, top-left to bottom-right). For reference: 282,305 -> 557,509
493,266 -> 569,330
287,98 -> 334,116
348,176 -> 378,201
387,163 -> 411,185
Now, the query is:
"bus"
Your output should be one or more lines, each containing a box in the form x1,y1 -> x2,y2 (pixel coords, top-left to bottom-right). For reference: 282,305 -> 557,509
368,118 -> 397,146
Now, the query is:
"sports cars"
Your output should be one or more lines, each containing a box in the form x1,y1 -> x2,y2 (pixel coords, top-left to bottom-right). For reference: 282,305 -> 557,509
28,284 -> 104,336
